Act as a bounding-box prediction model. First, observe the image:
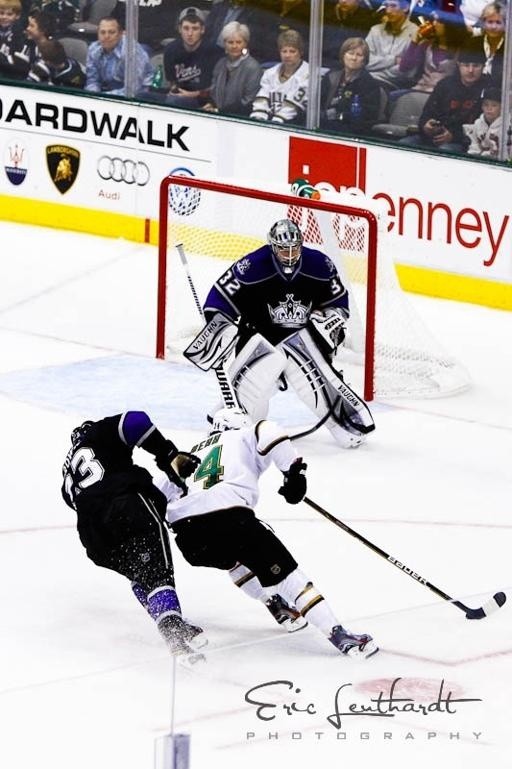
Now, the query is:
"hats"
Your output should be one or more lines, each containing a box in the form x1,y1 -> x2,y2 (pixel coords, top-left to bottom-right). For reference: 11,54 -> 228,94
180,6 -> 206,25
457,47 -> 486,64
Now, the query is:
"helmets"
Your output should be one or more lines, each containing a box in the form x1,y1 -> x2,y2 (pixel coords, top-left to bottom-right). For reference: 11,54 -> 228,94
209,408 -> 252,436
269,219 -> 302,265
69,420 -> 92,440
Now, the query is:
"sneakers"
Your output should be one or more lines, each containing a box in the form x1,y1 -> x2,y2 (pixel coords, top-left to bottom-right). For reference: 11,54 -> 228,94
161,614 -> 204,643
270,599 -> 304,628
328,624 -> 374,656
329,424 -> 367,449
158,621 -> 194,661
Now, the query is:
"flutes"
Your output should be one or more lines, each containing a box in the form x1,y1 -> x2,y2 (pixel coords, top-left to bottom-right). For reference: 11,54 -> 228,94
303,495 -> 506,620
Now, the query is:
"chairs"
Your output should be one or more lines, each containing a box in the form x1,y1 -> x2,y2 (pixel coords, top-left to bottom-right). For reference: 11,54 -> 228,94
57,38 -> 87,67
69,0 -> 118,39
150,55 -> 172,92
373,92 -> 431,138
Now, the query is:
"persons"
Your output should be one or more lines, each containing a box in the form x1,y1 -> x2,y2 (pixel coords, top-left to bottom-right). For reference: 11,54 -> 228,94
207,0 -> 266,61
459,0 -> 493,35
249,29 -> 310,125
160,407 -> 381,665
28,0 -> 76,39
318,37 -> 380,136
136,7 -> 213,112
201,22 -> 259,116
398,45 -> 496,159
460,88 -> 511,162
327,0 -> 371,31
82,15 -> 150,98
363,0 -> 421,89
203,218 -> 375,462
1,0 -> 25,80
13,12 -> 67,87
29,43 -> 85,88
389,8 -> 470,125
55,412 -> 207,662
457,4 -> 506,100
109,0 -> 176,46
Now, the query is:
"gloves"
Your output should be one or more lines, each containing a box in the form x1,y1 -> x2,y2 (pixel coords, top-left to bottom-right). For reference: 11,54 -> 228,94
276,460 -> 308,503
305,309 -> 349,356
157,440 -> 201,493
